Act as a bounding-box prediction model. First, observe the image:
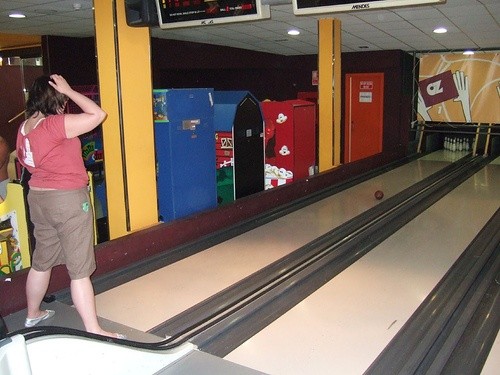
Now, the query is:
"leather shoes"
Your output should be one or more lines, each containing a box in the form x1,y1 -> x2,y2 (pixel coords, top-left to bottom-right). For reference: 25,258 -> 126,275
24,310 -> 57,328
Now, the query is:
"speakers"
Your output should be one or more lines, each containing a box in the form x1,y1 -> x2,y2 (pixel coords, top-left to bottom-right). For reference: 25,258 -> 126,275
124,0 -> 159,28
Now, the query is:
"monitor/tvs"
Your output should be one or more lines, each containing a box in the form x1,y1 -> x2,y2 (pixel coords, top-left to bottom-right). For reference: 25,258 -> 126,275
155,0 -> 262,29
292,0 -> 445,17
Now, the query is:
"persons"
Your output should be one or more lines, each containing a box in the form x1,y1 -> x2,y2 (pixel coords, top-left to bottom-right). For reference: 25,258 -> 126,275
17,72 -> 124,342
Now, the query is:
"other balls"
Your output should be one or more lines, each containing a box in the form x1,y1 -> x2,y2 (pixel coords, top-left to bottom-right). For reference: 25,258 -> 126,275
374,191 -> 384,200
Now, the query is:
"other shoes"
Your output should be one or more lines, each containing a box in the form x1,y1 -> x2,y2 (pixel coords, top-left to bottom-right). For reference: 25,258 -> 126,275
112,333 -> 126,339
